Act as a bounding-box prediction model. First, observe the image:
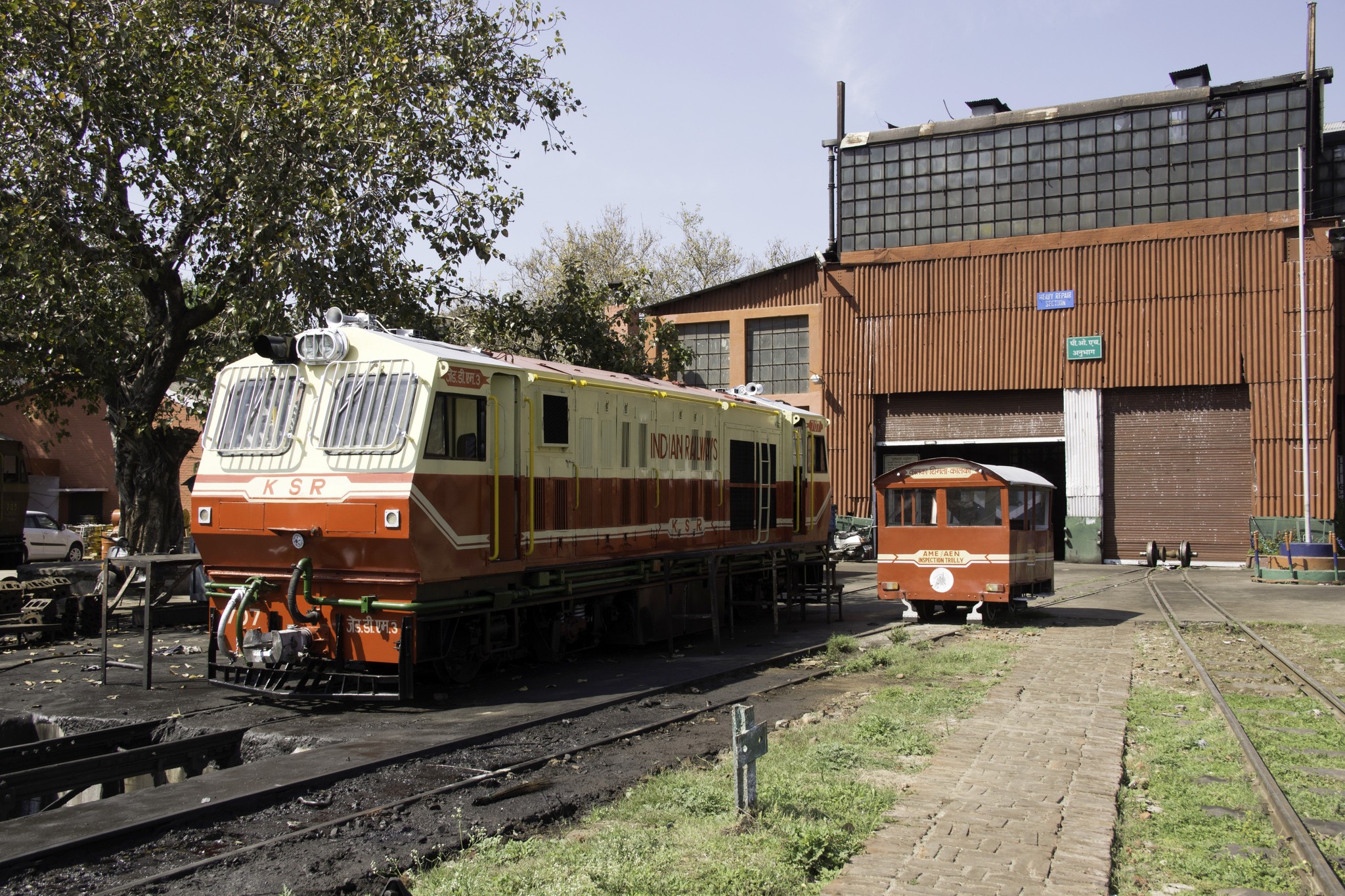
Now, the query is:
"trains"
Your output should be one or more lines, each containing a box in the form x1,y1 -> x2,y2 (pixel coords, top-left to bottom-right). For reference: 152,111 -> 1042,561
871,457 -> 1059,628
187,306 -> 838,710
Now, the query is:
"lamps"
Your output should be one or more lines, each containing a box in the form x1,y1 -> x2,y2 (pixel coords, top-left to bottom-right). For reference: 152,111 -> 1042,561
1324,227 -> 1345,243
814,251 -> 840,267
811,375 -> 824,385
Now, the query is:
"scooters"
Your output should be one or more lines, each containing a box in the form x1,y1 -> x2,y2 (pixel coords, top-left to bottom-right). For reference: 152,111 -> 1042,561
92,535 -> 178,605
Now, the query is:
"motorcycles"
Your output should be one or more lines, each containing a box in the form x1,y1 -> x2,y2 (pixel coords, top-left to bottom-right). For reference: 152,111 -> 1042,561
823,528 -> 873,563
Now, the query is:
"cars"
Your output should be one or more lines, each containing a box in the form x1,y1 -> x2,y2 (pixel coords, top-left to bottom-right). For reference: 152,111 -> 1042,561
22,510 -> 85,565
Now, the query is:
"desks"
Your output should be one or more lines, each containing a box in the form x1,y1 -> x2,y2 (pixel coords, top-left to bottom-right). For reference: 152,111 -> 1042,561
102,552 -> 202,690
664,539 -> 832,656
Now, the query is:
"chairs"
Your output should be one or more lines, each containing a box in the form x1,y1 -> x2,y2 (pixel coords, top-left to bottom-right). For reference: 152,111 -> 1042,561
456,433 -> 476,458
798,559 -> 845,624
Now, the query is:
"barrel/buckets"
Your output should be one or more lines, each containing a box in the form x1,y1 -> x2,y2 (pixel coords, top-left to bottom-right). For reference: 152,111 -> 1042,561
101,532 -> 118,560
111,510 -> 121,531
